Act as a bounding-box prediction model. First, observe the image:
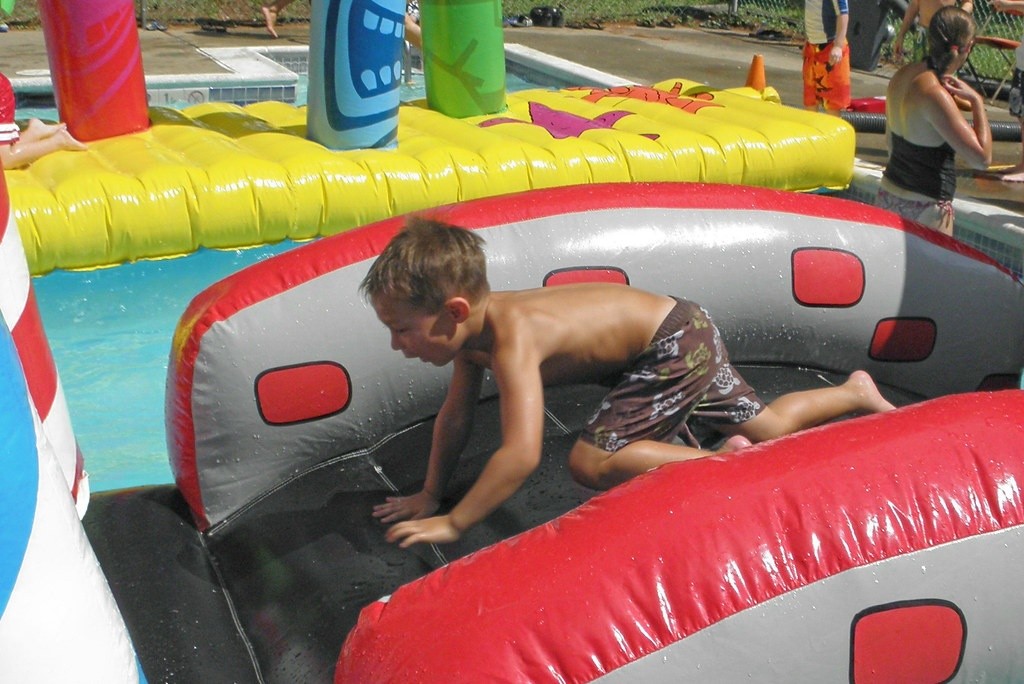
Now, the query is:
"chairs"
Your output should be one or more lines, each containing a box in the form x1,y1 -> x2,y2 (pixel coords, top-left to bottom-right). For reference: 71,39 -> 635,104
960,0 -> 1024,106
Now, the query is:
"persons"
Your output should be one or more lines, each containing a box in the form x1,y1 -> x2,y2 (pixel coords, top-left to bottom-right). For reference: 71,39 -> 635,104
802,1 -> 1023,240
404,0 -> 423,49
262,0 -> 294,38
1,72 -> 89,169
364,220 -> 899,549
